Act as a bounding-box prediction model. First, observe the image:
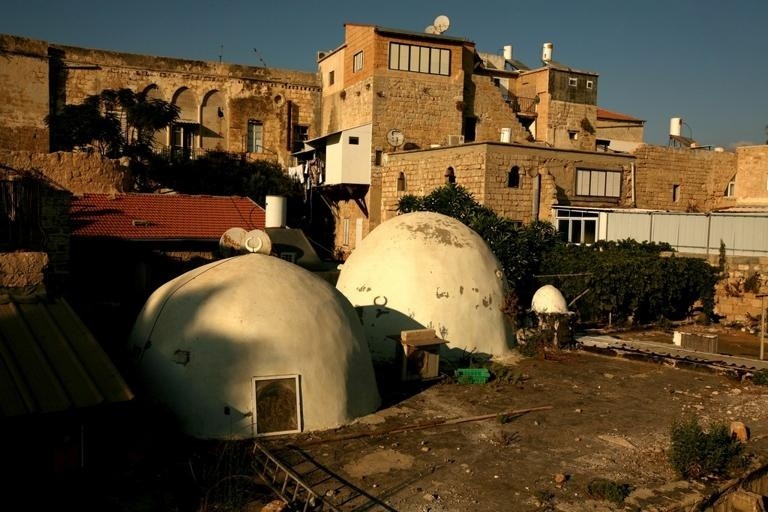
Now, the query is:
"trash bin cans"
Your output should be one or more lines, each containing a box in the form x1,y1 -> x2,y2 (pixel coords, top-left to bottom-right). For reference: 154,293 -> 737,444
555,312 -> 576,349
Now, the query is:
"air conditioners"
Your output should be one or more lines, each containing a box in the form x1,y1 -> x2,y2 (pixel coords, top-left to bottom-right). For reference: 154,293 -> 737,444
447,135 -> 464,147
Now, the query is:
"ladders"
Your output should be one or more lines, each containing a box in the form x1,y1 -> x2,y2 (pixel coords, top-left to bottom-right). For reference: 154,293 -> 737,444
249,442 -> 341,512
